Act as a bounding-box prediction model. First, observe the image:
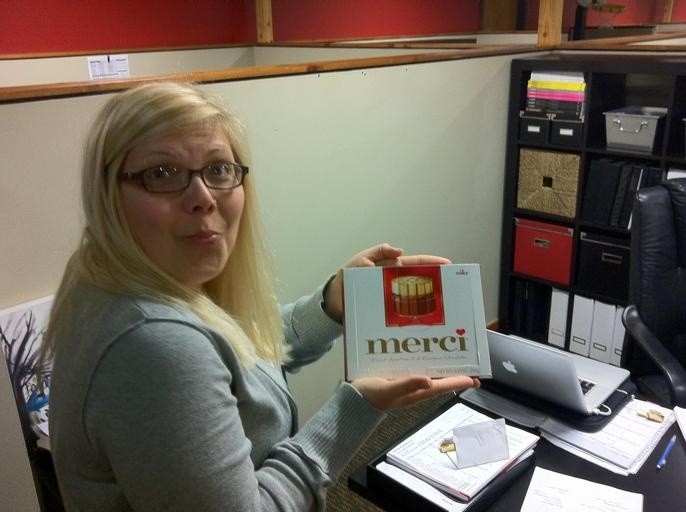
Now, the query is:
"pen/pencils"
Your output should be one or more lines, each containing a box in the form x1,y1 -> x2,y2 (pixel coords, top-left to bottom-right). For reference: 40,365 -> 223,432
657,434 -> 678,470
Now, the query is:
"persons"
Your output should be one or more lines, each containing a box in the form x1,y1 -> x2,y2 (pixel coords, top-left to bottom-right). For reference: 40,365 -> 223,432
38,83 -> 481,512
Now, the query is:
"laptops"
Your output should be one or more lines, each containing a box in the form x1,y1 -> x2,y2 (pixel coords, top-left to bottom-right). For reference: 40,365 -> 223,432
485,329 -> 630,415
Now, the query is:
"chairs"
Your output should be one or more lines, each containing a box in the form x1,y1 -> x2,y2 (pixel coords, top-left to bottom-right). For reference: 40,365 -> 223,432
620,187 -> 685,413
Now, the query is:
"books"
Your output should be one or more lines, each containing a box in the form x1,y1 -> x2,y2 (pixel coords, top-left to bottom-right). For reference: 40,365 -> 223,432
524,68 -> 585,120
386,404 -> 540,502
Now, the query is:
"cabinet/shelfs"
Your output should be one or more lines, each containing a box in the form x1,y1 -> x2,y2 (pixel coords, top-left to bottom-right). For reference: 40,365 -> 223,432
496,51 -> 685,371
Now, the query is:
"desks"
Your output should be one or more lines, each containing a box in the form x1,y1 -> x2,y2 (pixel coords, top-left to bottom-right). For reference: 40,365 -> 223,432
346,384 -> 685,512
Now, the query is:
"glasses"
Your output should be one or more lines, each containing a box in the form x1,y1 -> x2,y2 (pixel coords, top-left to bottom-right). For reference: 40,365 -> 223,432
119,160 -> 249,194
